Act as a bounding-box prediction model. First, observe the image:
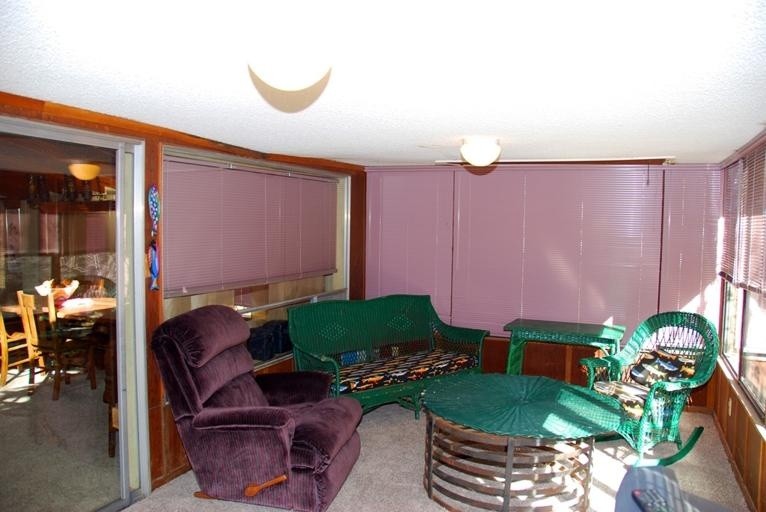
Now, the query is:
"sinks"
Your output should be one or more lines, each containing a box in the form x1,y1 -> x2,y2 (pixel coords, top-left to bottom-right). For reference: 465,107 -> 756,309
288,294 -> 490,421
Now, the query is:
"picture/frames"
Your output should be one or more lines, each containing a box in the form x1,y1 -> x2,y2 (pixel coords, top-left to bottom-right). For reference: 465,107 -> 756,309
505,319 -> 626,375
421,371 -> 624,511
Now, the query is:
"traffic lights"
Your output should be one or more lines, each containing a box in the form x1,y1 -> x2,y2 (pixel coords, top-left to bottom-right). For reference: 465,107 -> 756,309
630,349 -> 695,388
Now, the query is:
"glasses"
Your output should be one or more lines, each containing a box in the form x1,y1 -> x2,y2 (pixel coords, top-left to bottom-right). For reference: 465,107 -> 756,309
68,160 -> 101,182
460,141 -> 501,167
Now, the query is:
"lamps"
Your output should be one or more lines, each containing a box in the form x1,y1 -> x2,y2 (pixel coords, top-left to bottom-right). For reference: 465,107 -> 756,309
153,304 -> 362,512
0,275 -> 119,401
580,313 -> 721,470
